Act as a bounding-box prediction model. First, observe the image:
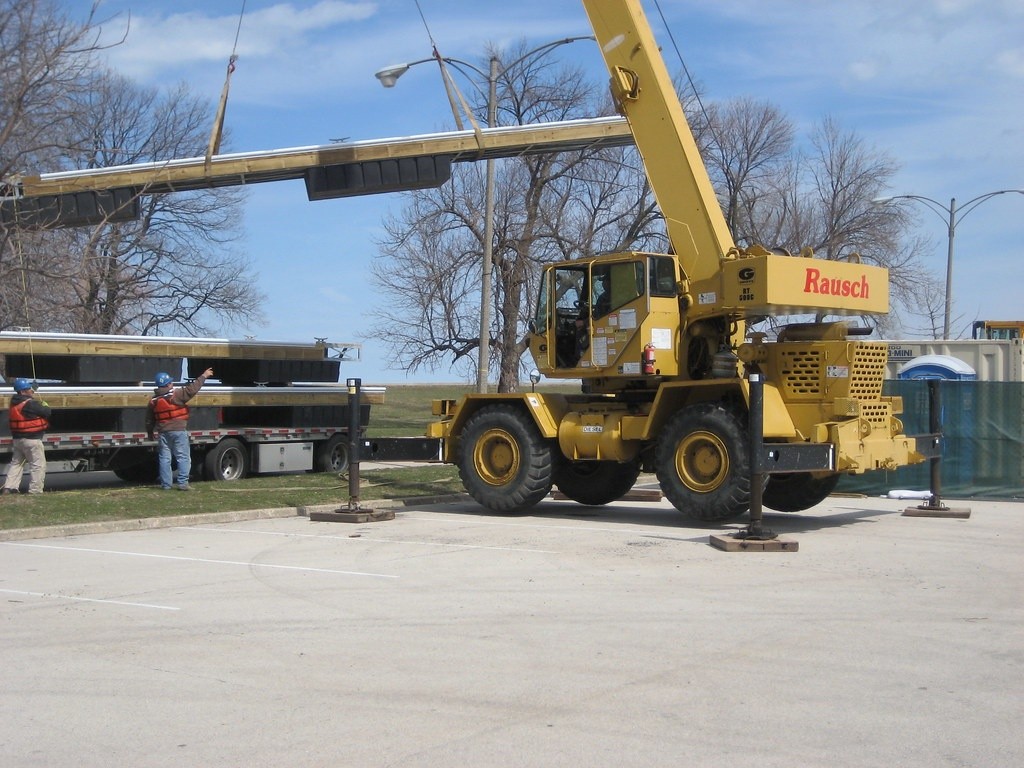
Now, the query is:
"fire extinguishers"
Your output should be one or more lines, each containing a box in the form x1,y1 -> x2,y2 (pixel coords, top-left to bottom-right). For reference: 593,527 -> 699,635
644,342 -> 657,374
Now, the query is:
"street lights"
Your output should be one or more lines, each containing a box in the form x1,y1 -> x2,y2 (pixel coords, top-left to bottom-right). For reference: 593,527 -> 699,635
873,190 -> 1024,339
374,36 -> 596,392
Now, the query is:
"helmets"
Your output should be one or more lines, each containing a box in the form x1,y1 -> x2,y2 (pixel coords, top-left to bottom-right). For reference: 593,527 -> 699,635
13,379 -> 33,391
155,372 -> 173,387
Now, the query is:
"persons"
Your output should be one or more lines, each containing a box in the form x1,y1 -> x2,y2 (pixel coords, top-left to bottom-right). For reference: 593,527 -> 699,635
2,378 -> 51,494
575,272 -> 611,328
145,368 -> 213,490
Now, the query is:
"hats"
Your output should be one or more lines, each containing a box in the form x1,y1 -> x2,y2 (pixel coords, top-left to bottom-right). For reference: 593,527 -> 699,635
598,272 -> 610,281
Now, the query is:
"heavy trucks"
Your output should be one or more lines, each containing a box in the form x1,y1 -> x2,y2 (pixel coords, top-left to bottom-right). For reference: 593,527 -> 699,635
1,427 -> 367,484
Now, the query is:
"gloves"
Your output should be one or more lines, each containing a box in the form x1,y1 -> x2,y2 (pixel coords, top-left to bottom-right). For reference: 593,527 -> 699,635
41,401 -> 48,406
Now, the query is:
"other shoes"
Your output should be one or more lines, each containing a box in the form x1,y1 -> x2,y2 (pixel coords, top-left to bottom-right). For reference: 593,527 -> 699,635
3,488 -> 17,494
28,492 -> 45,497
162,486 -> 171,490
562,358 -> 570,367
178,487 -> 196,490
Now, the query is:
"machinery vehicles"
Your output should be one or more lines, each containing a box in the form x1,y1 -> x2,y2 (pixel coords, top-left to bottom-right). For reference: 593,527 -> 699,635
0,0 -> 927,522
